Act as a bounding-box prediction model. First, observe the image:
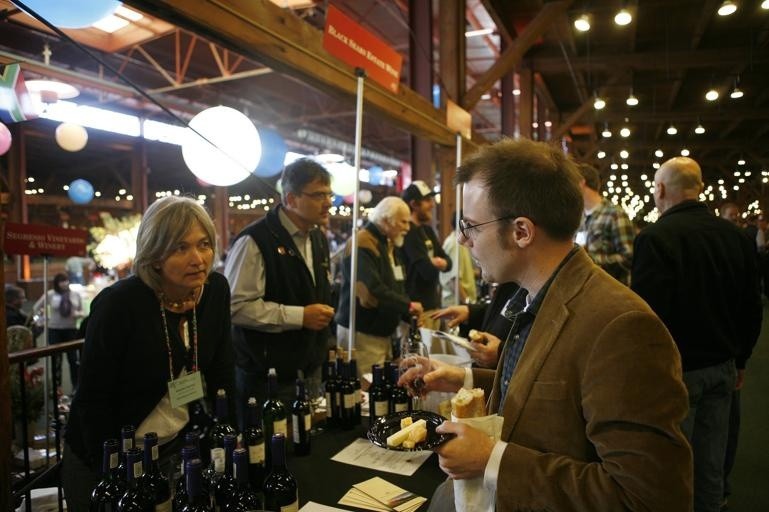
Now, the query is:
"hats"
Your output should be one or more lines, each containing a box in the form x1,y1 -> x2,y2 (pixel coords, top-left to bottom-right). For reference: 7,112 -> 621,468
404,181 -> 441,201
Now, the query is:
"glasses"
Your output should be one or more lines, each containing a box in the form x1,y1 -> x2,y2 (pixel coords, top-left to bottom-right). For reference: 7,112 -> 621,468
302,191 -> 334,199
459,217 -> 508,240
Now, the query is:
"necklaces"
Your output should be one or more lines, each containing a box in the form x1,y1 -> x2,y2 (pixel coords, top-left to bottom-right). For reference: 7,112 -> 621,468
162,287 -> 198,310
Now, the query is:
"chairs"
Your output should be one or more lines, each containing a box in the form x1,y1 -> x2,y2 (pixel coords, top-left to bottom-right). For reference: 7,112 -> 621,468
6,327 -> 45,425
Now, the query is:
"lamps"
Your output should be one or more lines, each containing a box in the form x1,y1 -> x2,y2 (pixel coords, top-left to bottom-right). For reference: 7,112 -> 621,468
18,43 -> 80,113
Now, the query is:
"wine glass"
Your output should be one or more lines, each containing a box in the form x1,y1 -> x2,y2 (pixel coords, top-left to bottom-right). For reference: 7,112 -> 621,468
399,341 -> 430,412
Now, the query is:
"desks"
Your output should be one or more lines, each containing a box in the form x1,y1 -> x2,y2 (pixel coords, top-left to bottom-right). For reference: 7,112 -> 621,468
157,412 -> 453,512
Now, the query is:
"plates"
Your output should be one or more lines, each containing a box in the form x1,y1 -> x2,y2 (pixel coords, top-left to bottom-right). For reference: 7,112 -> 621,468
368,410 -> 452,453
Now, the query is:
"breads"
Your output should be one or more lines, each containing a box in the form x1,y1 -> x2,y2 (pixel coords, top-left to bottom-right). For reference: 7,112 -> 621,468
469,329 -> 485,342
387,416 -> 427,448
452,387 -> 486,417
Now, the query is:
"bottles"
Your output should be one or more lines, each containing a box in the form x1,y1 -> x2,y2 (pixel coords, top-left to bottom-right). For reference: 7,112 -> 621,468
367,361 -> 413,422
91,345 -> 362,511
33,307 -> 44,323
406,316 -> 422,354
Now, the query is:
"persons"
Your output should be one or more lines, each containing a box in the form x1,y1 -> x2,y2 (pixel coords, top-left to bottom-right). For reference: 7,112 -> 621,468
720,204 -> 739,224
1,274 -> 86,398
388,134 -> 695,512
578,163 -> 637,282
340,180 -> 477,377
630,152 -> 769,512
224,158 -> 337,377
60,192 -> 237,511
